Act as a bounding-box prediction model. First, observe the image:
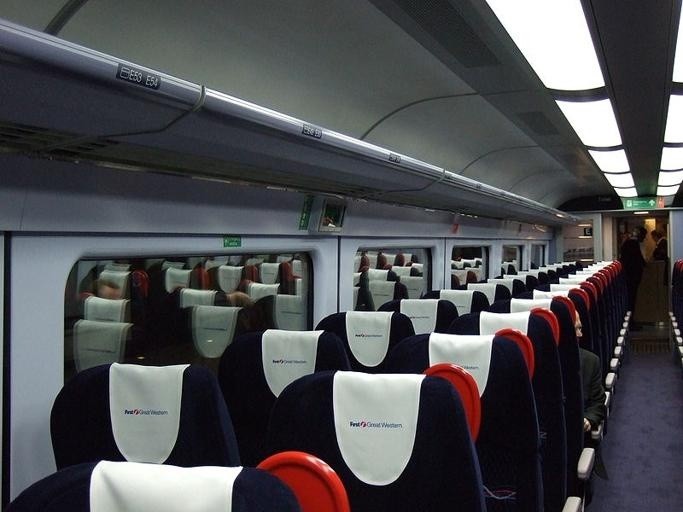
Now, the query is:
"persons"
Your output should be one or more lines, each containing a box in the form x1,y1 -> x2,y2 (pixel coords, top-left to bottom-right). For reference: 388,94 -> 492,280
651,230 -> 668,287
226,292 -> 251,331
574,309 -> 607,433
86,279 -> 123,299
621,226 -> 647,332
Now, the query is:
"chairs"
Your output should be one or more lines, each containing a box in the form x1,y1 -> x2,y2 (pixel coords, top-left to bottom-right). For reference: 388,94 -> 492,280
1,250 -> 632,512
668,257 -> 682,367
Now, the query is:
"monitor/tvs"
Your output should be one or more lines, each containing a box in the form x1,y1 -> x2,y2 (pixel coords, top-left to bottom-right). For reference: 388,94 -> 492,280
308,195 -> 349,235
